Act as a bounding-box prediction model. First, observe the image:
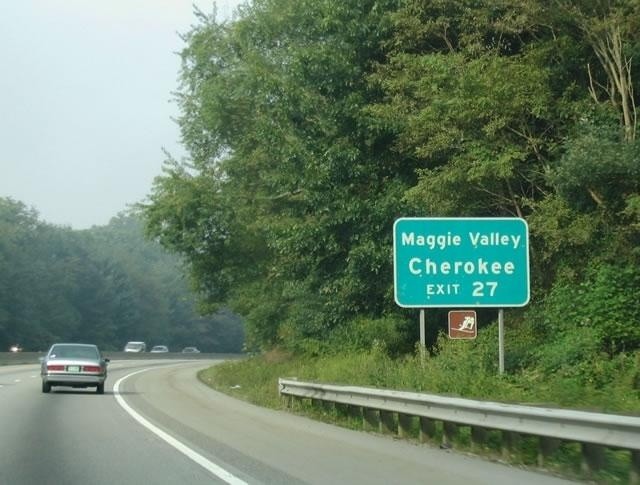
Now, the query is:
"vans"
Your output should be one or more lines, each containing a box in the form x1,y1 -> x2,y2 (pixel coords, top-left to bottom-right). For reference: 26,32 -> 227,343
124,341 -> 146,354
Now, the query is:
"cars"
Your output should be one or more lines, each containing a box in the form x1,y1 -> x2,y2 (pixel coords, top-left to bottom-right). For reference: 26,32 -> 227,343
182,347 -> 201,354
38,342 -> 109,396
150,346 -> 169,353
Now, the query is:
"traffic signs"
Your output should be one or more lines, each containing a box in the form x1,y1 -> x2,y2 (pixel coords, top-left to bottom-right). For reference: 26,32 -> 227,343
394,217 -> 530,307
